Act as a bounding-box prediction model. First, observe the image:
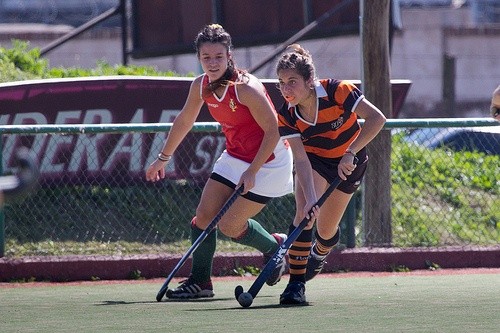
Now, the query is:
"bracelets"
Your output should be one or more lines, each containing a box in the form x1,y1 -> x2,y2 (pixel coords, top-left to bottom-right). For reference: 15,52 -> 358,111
344,149 -> 357,157
158,152 -> 172,162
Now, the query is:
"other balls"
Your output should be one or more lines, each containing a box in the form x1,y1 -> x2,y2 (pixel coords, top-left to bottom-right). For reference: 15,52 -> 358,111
239,292 -> 251,307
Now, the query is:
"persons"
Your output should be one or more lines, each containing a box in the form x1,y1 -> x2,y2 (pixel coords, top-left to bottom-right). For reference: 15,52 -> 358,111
278,44 -> 386,307
145,24 -> 294,300
491,86 -> 500,121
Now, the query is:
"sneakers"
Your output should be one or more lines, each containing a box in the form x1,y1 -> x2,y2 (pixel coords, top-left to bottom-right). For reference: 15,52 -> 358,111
305,241 -> 331,281
279,280 -> 306,305
166,275 -> 215,300
262,233 -> 288,286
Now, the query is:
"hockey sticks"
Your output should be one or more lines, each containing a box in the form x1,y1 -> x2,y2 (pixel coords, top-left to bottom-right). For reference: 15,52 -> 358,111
234,156 -> 361,308
154,184 -> 244,303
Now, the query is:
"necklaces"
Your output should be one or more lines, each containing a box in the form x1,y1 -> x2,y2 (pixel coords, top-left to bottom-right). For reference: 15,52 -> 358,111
298,95 -> 313,120
